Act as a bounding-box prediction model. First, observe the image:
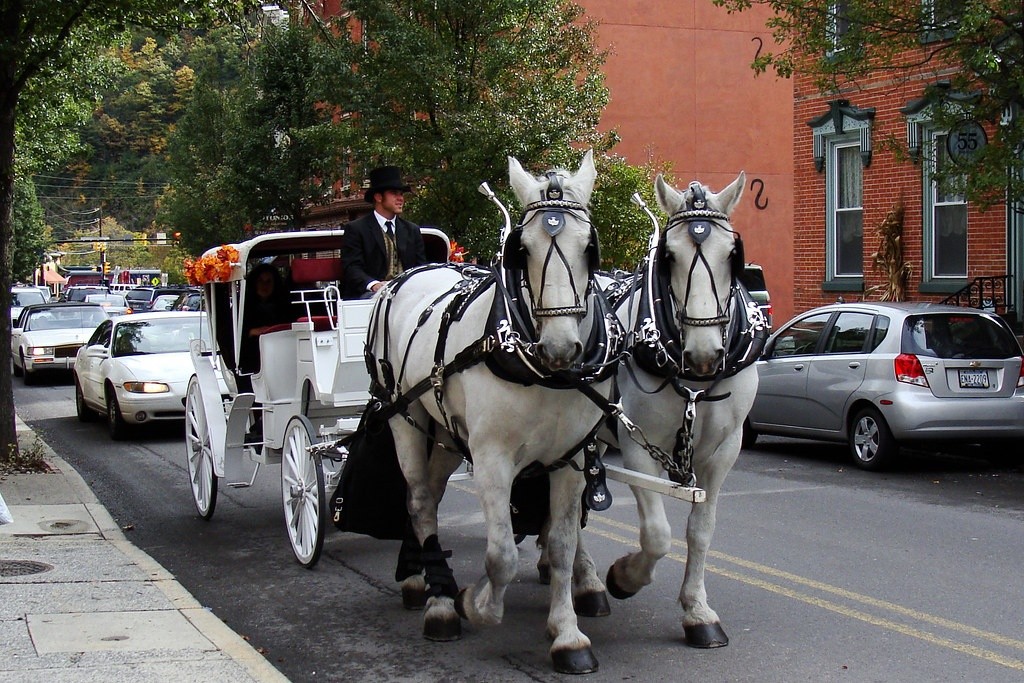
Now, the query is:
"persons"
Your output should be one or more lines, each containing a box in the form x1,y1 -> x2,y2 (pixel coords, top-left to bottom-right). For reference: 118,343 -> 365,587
225,264 -> 299,433
340,166 -> 426,300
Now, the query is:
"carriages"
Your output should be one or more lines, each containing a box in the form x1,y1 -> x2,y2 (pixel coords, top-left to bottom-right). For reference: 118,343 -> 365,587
183,146 -> 761,675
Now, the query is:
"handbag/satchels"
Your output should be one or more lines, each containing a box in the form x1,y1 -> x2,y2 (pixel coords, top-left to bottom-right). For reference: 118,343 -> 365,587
329,397 -> 410,541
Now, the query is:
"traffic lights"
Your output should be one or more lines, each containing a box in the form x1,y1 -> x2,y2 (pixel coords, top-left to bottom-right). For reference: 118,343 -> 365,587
105,262 -> 111,275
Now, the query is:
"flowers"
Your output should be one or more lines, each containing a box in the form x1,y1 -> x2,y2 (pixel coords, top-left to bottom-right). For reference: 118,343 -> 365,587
450,239 -> 465,262
186,244 -> 239,286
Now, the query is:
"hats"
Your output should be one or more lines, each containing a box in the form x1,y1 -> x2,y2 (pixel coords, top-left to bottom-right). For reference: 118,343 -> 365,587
364,166 -> 411,204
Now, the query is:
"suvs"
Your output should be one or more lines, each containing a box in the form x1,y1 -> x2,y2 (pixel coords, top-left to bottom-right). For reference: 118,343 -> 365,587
740,261 -> 772,337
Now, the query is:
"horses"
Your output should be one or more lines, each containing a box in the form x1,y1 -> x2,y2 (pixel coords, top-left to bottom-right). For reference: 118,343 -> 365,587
537,170 -> 762,647
365,148 -> 619,674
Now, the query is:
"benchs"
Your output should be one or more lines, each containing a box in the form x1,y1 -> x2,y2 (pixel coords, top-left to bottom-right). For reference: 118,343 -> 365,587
290,258 -> 343,332
250,322 -> 294,403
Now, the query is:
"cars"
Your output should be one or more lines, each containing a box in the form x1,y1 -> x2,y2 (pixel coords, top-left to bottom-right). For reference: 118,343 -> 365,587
6,281 -> 209,336
72,311 -> 237,441
738,299 -> 1024,469
11,301 -> 111,385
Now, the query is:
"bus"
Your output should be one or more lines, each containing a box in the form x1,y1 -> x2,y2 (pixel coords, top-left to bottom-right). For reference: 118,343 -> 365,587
62,269 -> 168,295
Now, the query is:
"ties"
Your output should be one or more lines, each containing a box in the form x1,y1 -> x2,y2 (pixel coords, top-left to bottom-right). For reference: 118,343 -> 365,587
385,221 -> 394,242
